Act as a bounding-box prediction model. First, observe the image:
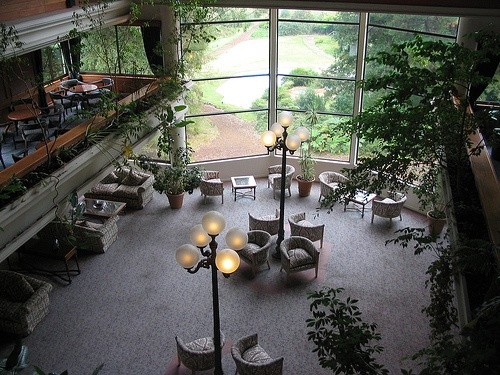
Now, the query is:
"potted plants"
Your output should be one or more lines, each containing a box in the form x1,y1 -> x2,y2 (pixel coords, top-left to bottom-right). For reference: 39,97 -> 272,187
154,144 -> 200,212
426,171 -> 449,237
293,107 -> 324,198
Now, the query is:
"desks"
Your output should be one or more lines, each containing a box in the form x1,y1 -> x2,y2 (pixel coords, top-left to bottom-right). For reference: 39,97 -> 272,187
343,189 -> 377,218
229,175 -> 258,202
68,83 -> 98,110
7,108 -> 42,137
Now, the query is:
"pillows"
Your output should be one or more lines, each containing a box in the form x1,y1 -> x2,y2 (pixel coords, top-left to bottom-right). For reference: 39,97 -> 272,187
121,169 -> 142,186
101,170 -> 118,184
86,221 -> 103,228
0,269 -> 35,300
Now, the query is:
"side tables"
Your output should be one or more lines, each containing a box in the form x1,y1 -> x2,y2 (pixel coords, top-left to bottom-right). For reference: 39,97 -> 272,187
35,242 -> 81,284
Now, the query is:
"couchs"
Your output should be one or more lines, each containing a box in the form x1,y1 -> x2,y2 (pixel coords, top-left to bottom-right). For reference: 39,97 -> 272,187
83,167 -> 155,210
0,266 -> 54,338
51,214 -> 121,254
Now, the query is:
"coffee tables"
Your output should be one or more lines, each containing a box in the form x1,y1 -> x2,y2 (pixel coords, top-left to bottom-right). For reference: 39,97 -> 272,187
76,197 -> 128,218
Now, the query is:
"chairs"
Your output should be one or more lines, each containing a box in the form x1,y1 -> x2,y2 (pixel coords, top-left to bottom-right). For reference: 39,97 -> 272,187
368,190 -> 408,227
0,75 -> 116,171
175,327 -> 226,375
266,164 -> 296,199
243,208 -> 280,231
237,229 -> 273,271
230,330 -> 286,375
288,209 -> 326,249
278,236 -> 322,282
317,168 -> 349,204
196,169 -> 225,205
